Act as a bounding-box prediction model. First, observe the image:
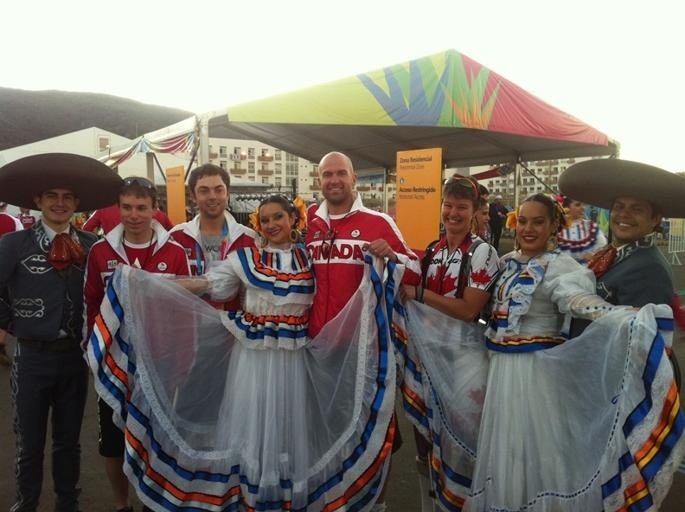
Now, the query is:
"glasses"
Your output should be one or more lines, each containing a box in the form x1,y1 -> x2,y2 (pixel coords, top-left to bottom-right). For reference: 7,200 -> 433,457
444,176 -> 475,190
120,175 -> 155,190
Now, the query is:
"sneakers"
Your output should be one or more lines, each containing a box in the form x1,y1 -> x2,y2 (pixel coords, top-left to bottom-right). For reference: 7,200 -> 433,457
0,345 -> 13,366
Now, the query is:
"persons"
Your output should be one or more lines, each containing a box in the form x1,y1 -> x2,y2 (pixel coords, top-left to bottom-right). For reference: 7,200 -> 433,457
304,150 -> 423,512
80,177 -> 196,511
82,203 -> 174,235
0,201 -> 25,366
117,193 -> 314,501
16,207 -> 35,229
167,164 -> 258,450
0,152 -> 118,512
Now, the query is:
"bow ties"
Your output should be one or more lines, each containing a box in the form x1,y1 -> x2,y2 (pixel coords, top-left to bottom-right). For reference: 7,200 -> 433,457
49,233 -> 86,269
584,246 -> 617,278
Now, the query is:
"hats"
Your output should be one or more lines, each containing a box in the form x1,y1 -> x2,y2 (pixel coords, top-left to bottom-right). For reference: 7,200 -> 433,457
494,194 -> 503,200
0,151 -> 124,213
555,156 -> 685,222
